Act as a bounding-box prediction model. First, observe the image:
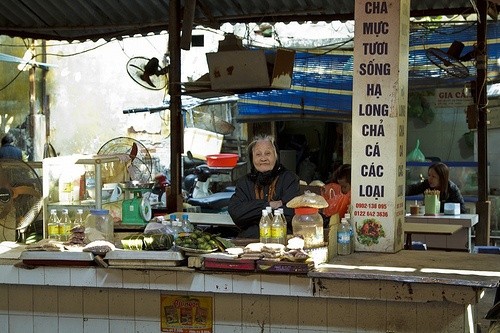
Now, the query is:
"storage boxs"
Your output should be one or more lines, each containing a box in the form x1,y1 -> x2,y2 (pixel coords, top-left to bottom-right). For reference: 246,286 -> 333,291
182,127 -> 224,162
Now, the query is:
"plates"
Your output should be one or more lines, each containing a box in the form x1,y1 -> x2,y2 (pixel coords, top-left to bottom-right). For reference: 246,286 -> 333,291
174,244 -> 218,252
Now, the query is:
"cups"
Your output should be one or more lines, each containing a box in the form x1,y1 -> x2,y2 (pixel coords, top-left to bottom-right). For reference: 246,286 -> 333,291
103,183 -> 122,203
410,207 -> 419,216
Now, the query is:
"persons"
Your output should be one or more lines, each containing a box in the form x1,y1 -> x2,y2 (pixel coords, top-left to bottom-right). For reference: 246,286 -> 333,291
406,161 -> 476,236
227,133 -> 300,239
298,149 -> 321,184
0,134 -> 23,160
323,162 -> 351,194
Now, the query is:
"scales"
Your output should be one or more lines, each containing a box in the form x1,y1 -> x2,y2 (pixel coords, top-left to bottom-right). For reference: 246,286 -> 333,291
119,182 -> 154,225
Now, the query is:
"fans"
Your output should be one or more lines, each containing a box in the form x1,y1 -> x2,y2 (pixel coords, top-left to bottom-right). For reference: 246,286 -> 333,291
97,137 -> 152,182
126,56 -> 170,91
0,158 -> 44,242
426,40 -> 477,79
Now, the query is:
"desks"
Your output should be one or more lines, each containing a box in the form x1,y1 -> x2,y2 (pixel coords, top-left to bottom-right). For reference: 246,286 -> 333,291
163,212 -> 235,235
404,214 -> 479,252
403,222 -> 464,251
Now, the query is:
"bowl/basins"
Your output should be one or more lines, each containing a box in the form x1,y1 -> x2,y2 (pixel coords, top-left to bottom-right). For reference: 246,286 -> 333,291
205,154 -> 239,167
88,189 -> 113,201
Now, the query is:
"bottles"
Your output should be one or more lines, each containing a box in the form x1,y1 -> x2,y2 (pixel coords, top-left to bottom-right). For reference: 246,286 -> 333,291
83,209 -> 115,244
72,208 -> 84,227
58,208 -> 72,242
337,213 -> 355,255
48,208 -> 60,242
162,214 -> 193,249
291,207 -> 327,250
259,206 -> 288,245
